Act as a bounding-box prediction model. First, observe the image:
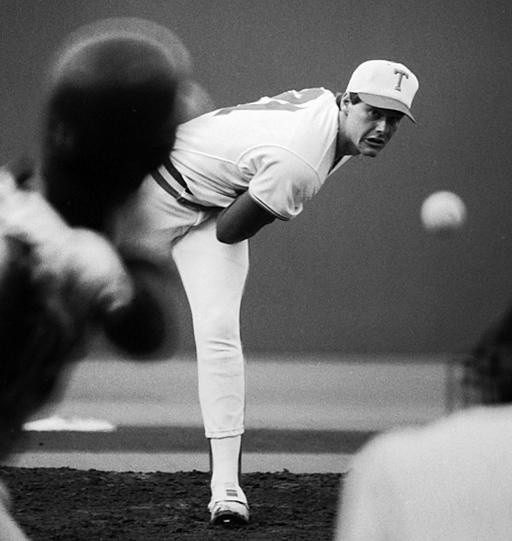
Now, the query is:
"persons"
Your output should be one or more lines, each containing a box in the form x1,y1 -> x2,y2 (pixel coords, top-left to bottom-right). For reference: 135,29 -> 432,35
332,310 -> 512,541
113,57 -> 419,528
2,29 -> 179,541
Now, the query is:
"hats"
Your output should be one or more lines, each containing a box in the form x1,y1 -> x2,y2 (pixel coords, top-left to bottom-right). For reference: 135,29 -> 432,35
346,60 -> 419,123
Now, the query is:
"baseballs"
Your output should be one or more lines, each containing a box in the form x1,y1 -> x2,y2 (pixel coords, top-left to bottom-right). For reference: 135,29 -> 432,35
420,192 -> 465,234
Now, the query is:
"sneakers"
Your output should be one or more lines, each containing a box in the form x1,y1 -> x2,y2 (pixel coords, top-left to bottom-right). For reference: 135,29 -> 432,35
208,482 -> 251,528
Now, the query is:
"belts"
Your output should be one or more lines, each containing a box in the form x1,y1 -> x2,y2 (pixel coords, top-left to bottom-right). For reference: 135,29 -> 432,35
150,159 -> 225,212
